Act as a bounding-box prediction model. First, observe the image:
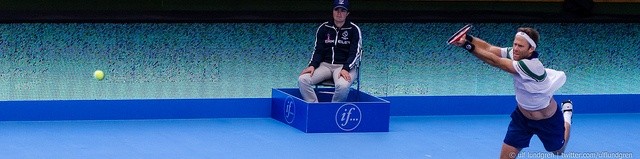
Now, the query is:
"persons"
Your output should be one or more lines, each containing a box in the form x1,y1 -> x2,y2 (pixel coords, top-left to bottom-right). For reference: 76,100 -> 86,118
298,0 -> 363,103
455,28 -> 573,159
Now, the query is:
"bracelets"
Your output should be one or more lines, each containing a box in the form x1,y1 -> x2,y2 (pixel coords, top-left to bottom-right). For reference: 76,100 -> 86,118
466,34 -> 473,42
462,41 -> 475,53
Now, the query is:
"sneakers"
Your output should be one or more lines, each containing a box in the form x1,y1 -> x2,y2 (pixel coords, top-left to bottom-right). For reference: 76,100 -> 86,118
561,100 -> 574,112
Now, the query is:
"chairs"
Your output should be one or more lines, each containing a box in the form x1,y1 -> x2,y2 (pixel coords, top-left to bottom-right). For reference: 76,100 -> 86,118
315,47 -> 363,102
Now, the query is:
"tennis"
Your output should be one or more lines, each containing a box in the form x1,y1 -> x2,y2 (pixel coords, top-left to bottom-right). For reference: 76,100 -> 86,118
94,70 -> 105,81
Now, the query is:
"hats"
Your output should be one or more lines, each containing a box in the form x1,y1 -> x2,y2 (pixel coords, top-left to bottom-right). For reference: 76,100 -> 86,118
333,0 -> 350,11
